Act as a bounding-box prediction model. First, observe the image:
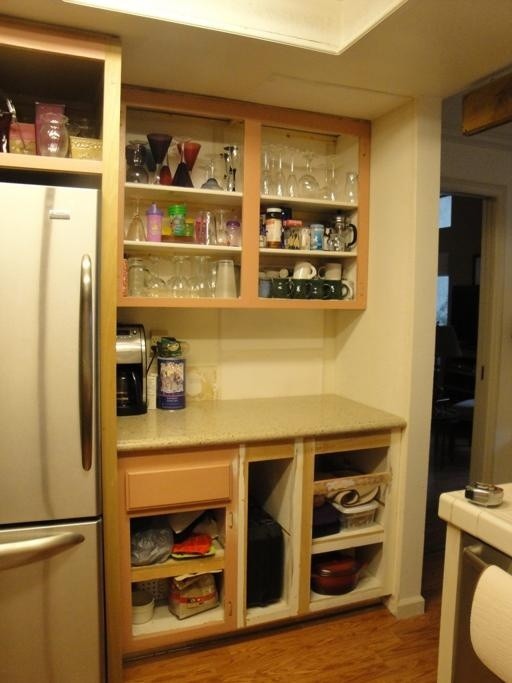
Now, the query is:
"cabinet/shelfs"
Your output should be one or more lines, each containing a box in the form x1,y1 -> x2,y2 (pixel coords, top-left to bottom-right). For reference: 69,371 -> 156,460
115,450 -> 245,653
122,83 -> 370,311
306,435 -> 402,609
0,44 -> 108,174
242,444 -> 300,623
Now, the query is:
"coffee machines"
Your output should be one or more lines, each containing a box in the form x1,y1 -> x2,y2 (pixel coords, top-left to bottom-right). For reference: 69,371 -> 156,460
115,322 -> 149,415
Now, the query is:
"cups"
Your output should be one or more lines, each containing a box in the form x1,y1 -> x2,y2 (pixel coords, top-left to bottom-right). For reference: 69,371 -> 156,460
123,251 -> 351,302
124,195 -> 242,247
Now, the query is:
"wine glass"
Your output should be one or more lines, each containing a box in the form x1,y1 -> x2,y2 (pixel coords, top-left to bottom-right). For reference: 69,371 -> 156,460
124,130 -> 360,204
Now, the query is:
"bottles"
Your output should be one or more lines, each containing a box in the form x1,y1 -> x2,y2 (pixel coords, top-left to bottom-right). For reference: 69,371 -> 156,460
38,111 -> 69,159
259,211 -> 356,250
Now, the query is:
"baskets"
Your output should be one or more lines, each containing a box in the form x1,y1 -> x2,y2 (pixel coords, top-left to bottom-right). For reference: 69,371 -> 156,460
135,577 -> 169,601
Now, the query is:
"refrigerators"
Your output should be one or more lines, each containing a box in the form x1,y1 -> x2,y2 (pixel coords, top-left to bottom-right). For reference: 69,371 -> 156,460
0,181 -> 108,683
